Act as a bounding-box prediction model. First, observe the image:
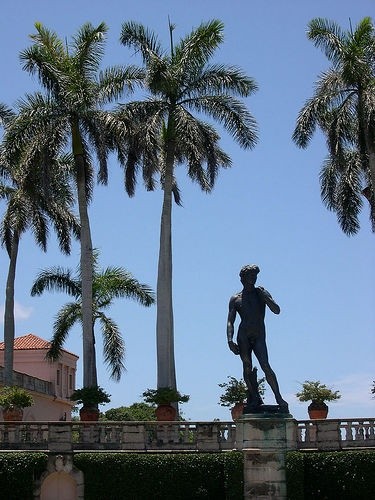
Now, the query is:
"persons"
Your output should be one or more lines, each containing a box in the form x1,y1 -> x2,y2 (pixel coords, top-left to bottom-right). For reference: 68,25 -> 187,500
226,264 -> 289,413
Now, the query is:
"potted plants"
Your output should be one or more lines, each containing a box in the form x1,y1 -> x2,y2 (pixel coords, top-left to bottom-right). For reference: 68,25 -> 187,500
66,384 -> 111,421
0,385 -> 34,422
216,375 -> 267,422
296,379 -> 342,420
143,386 -> 190,421
